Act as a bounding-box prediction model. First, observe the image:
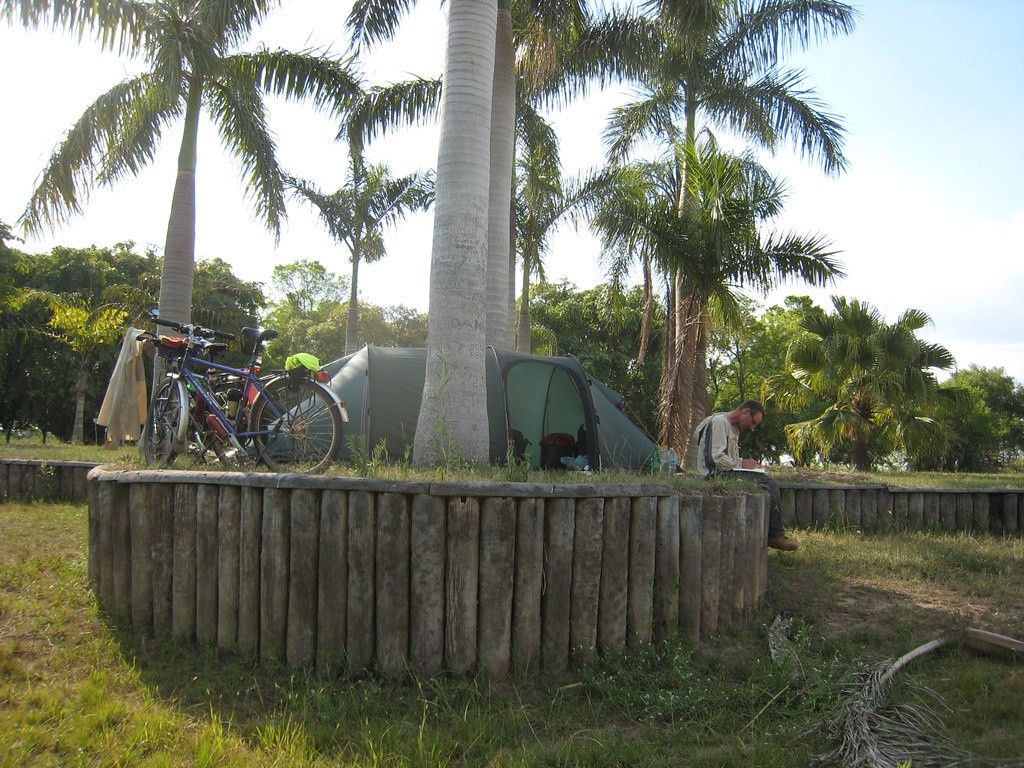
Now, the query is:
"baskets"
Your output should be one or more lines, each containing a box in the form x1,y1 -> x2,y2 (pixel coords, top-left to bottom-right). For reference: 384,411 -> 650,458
160,344 -> 211,379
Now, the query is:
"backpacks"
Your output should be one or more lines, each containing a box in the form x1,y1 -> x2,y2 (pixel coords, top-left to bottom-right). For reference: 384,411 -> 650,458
539,432 -> 576,471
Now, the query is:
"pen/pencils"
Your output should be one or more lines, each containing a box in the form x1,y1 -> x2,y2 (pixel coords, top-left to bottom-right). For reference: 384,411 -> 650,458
745,450 -> 754,460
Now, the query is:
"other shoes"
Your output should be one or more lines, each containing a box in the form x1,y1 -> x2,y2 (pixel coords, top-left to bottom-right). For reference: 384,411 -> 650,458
767,535 -> 799,551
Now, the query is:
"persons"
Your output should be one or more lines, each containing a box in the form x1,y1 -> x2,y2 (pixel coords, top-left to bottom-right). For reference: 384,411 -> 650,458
692,399 -> 798,551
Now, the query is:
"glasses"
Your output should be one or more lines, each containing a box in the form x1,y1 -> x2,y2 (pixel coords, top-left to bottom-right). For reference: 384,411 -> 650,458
750,413 -> 760,432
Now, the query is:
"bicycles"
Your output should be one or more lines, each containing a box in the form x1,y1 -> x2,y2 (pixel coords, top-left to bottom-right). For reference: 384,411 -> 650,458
143,318 -> 349,475
137,331 -> 277,470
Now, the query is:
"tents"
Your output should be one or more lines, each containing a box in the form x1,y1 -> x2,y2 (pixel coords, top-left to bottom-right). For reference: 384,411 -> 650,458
212,342 -> 687,478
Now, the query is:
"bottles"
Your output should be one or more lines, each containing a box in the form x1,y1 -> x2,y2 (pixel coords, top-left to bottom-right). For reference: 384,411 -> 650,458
228,389 -> 239,420
204,411 -> 228,440
195,391 -> 204,422
651,448 -> 676,473
